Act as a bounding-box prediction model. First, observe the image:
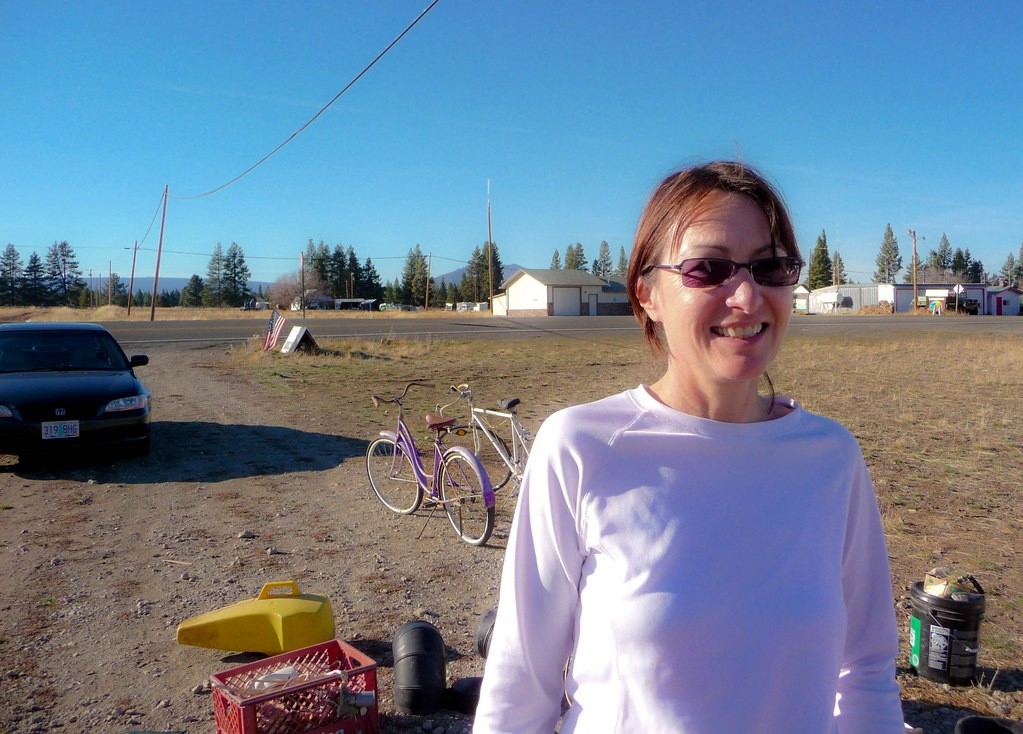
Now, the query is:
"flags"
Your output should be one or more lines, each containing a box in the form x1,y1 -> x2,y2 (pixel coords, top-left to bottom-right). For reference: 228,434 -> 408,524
263,310 -> 287,351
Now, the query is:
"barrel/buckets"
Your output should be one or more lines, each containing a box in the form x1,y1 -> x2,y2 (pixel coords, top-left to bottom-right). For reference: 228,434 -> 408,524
909,581 -> 987,685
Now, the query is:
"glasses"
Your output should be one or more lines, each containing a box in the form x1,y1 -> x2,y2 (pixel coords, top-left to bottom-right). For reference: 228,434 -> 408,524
640,256 -> 805,288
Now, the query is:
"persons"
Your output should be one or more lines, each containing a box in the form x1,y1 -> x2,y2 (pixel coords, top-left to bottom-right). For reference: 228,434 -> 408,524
472,143 -> 907,734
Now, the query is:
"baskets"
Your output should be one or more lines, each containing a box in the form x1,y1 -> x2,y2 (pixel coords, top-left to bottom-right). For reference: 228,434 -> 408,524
209,639 -> 379,734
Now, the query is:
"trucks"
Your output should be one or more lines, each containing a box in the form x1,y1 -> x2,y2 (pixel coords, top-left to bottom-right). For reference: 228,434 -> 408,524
917,295 -> 981,315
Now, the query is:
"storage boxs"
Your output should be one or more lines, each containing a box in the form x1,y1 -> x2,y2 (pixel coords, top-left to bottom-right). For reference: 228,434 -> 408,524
211,638 -> 380,734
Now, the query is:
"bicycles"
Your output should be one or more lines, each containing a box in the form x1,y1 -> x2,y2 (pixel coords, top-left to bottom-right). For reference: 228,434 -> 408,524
448,392 -> 538,510
366,382 -> 496,547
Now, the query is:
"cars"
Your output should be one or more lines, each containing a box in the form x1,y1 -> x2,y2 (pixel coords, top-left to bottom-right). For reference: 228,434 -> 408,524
0,322 -> 153,459
